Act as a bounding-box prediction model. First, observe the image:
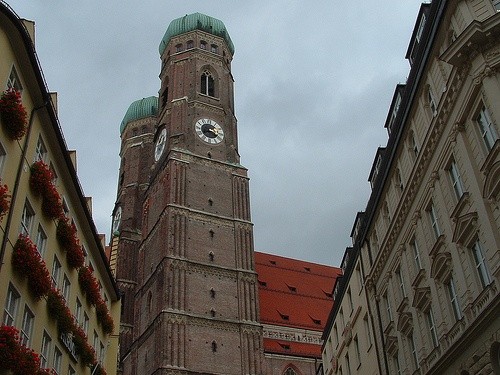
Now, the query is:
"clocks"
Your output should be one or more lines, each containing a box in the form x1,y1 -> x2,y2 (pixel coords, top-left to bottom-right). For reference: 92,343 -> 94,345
193,115 -> 226,147
153,128 -> 166,160
